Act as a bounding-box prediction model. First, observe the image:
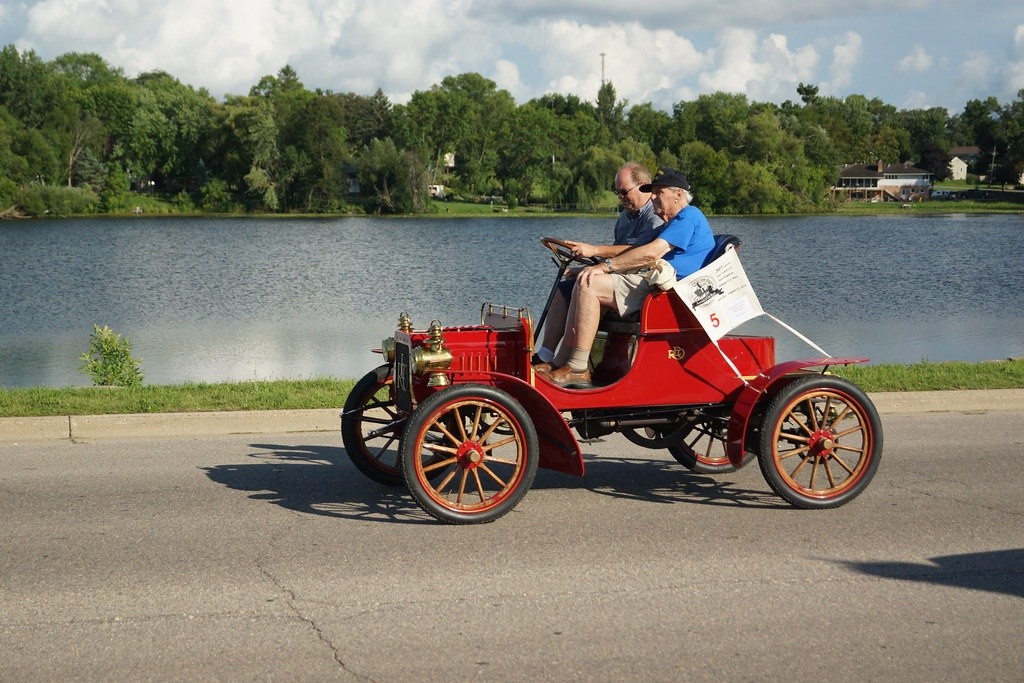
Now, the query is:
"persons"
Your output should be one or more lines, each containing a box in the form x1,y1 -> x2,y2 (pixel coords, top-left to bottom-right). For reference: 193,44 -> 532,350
534,169 -> 716,388
530,161 -> 666,365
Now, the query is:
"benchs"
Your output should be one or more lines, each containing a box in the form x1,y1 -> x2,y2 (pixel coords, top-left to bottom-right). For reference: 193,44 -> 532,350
602,234 -> 742,323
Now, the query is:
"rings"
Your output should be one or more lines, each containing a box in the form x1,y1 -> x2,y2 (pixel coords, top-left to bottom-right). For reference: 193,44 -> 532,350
576,253 -> 578,255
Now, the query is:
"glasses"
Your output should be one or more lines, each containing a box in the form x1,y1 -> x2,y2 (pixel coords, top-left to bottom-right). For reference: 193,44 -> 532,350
610,183 -> 639,195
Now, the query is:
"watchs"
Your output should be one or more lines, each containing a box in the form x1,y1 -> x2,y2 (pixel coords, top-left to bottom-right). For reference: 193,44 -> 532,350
605,258 -> 612,269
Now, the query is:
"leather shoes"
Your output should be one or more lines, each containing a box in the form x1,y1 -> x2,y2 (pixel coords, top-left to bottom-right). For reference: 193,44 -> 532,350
533,362 -> 593,388
532,352 -> 544,364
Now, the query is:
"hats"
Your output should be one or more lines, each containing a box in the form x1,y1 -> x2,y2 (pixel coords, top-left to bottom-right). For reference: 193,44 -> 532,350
638,167 -> 691,193
